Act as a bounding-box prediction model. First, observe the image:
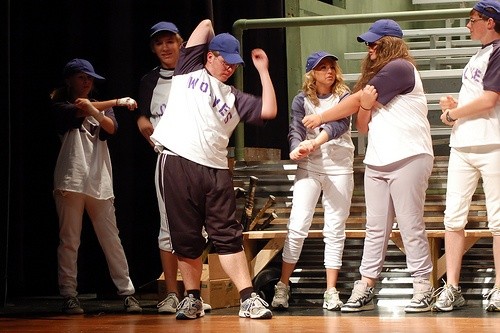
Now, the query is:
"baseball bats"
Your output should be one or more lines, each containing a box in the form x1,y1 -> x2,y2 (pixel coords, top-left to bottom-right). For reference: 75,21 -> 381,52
258,213 -> 278,230
240,175 -> 259,232
249,194 -> 276,231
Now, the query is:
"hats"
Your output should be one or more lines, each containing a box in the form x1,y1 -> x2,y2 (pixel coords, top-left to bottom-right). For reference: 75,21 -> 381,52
305,52 -> 339,72
208,33 -> 245,65
473,0 -> 500,24
65,59 -> 105,80
357,19 -> 403,43
148,22 -> 179,38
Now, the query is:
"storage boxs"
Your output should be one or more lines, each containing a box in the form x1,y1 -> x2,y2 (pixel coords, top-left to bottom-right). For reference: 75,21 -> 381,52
157,254 -> 240,308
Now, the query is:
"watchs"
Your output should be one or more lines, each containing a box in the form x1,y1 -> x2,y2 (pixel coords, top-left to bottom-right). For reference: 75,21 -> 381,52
446,111 -> 458,122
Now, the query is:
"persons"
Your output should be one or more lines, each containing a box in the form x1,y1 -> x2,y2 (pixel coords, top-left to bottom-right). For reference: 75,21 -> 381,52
134,21 -> 210,315
431,0 -> 500,312
272,51 -> 355,311
150,19 -> 277,319
302,19 -> 439,313
47,59 -> 142,314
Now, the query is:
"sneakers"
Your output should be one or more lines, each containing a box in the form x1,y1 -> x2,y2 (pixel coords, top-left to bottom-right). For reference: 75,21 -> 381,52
404,287 -> 436,313
124,296 -> 143,313
63,296 -> 84,314
341,280 -> 375,312
157,293 -> 180,315
483,287 -> 500,312
175,294 -> 205,320
271,281 -> 290,309
430,278 -> 466,312
322,287 -> 343,311
238,292 -> 273,319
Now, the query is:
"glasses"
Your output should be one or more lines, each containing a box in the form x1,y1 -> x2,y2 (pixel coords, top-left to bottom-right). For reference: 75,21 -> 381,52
216,56 -> 240,70
470,18 -> 488,25
365,42 -> 374,46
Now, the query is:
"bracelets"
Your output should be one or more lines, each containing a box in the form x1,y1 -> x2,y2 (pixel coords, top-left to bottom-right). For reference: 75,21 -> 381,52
115,98 -> 118,106
360,106 -> 371,110
319,114 -> 323,124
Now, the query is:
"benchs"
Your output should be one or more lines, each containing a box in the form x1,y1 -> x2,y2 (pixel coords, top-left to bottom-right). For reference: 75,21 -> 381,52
232,156 -> 500,295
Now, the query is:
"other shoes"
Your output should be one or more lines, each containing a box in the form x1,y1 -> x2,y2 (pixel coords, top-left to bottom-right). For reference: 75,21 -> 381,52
203,303 -> 212,313
